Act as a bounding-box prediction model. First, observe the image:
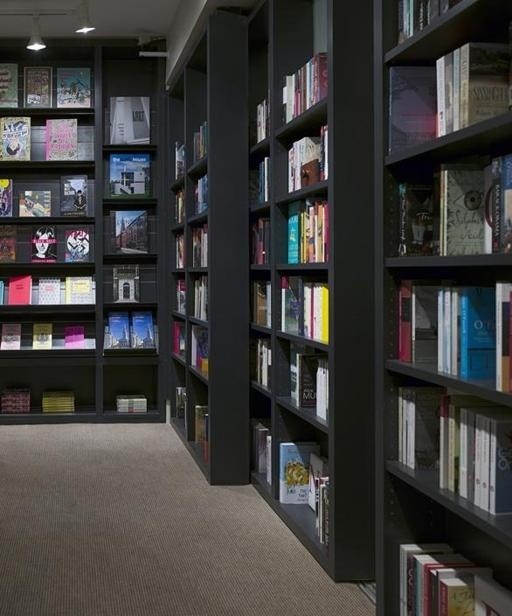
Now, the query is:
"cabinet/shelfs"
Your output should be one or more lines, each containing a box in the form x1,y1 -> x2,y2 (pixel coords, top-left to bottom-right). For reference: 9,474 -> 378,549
1,39 -> 167,425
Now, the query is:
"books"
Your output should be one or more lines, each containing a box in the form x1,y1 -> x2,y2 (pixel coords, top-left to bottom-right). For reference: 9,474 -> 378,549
1,64 -> 91,161
104,311 -> 159,349
110,211 -> 148,303
173,120 -> 209,462
389,0 -> 512,155
400,543 -> 512,615
399,387 -> 512,515
1,175 -> 89,263
117,395 -> 147,412
1,388 -> 75,412
1,273 -> 96,349
109,97 -> 150,196
252,53 -> 330,547
399,154 -> 512,392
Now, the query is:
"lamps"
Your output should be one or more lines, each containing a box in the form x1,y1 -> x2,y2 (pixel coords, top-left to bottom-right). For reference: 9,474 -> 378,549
0,1 -> 95,52
